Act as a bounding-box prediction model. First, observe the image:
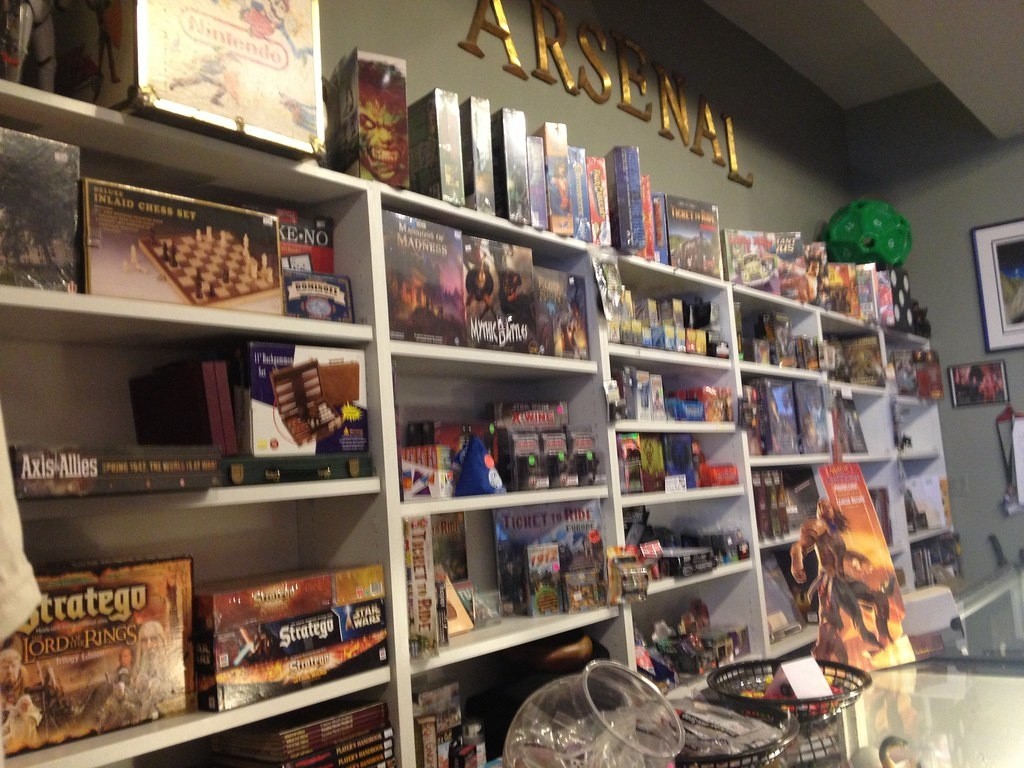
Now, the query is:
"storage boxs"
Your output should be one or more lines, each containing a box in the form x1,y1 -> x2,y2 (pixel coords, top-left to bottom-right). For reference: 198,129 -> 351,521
0,0 -> 916,754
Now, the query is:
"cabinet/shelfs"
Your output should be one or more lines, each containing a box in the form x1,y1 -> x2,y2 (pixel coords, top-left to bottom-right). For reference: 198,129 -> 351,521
0,78 -> 960,768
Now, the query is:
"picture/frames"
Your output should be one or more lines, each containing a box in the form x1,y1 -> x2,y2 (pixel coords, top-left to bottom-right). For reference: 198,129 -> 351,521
970,216 -> 1024,355
944,359 -> 1012,409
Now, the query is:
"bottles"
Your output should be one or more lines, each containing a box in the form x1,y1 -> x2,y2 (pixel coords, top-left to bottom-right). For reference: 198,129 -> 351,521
502,658 -> 685,768
